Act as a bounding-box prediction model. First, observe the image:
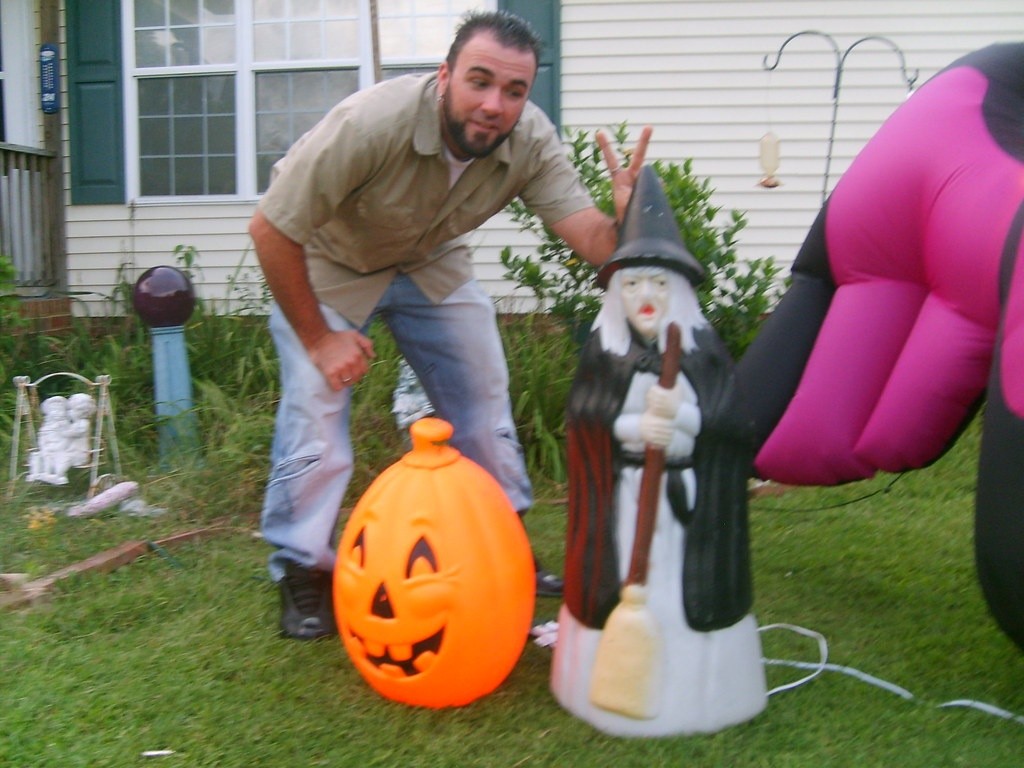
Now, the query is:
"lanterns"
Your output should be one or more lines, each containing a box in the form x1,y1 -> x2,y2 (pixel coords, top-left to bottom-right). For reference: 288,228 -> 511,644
332,418 -> 537,709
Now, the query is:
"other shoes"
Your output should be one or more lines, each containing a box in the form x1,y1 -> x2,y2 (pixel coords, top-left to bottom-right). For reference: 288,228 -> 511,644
535,567 -> 566,596
279,574 -> 336,639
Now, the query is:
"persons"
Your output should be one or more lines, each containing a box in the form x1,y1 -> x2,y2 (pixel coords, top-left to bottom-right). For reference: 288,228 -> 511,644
549,166 -> 768,736
390,357 -> 435,452
25,393 -> 97,485
248,10 -> 653,641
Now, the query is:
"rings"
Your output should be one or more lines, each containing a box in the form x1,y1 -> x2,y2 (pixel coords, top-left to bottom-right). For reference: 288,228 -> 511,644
342,376 -> 352,383
